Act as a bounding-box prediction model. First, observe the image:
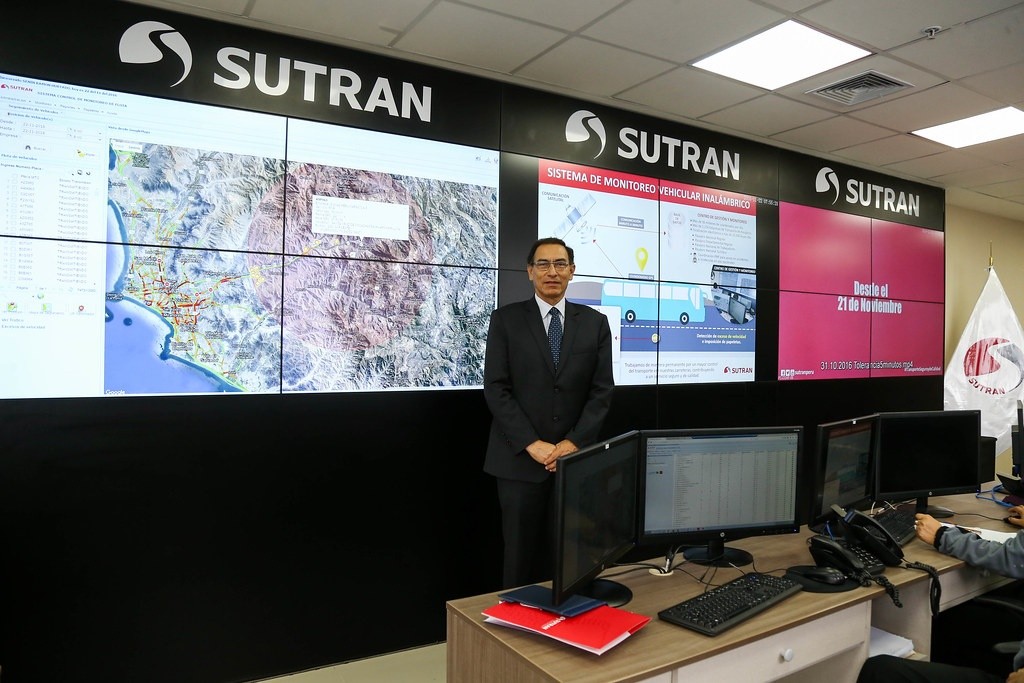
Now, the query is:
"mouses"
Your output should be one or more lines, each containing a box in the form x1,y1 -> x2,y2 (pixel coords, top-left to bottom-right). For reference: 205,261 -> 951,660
1004,513 -> 1020,524
803,566 -> 845,585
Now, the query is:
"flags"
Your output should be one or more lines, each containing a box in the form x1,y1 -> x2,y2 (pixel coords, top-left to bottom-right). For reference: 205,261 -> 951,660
944,266 -> 1024,457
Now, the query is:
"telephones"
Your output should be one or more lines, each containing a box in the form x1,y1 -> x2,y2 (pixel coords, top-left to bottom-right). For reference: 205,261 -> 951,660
806,533 -> 886,580
838,507 -> 904,567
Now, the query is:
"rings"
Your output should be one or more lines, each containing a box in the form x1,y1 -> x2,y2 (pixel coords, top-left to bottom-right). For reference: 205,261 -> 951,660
914,520 -> 918,530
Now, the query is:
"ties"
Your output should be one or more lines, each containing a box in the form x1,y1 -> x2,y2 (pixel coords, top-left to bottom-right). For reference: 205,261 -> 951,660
547,307 -> 562,369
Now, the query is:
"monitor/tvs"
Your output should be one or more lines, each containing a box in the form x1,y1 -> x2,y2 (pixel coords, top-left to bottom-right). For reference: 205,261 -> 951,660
808,413 -> 881,535
711,287 -> 756,324
550,430 -> 642,605
638,425 -> 805,568
1011,399 -> 1024,479
875,410 -> 981,518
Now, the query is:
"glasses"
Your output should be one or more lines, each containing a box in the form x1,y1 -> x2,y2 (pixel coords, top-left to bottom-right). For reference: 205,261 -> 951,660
531,261 -> 570,269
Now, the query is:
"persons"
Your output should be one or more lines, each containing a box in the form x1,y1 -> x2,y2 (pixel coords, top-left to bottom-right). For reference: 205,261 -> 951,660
915,505 -> 1024,579
483,238 -> 614,589
856,654 -> 1024,683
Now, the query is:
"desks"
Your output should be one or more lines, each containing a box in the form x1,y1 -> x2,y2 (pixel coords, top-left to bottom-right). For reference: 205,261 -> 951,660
809,486 -> 1024,616
445,525 -> 935,683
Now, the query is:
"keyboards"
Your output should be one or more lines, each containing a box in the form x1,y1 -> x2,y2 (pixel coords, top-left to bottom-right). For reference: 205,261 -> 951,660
658,572 -> 803,637
872,509 -> 919,546
